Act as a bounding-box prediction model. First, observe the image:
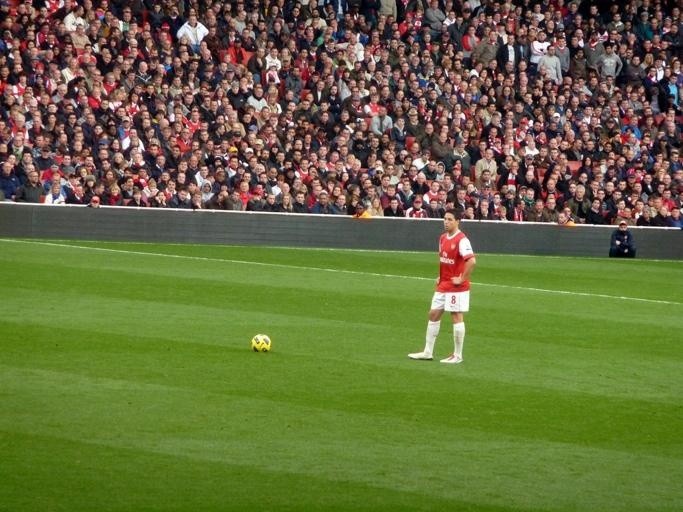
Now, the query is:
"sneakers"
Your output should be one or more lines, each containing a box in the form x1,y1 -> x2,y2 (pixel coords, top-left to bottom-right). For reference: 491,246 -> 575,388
408,351 -> 433,360
440,354 -> 463,363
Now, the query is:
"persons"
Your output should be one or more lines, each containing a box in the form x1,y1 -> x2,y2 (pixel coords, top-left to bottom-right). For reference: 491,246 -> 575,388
608,220 -> 636,258
0,0 -> 683,227
407,209 -> 476,365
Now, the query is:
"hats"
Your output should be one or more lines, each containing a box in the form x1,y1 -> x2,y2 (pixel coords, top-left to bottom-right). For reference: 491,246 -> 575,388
35,63 -> 263,154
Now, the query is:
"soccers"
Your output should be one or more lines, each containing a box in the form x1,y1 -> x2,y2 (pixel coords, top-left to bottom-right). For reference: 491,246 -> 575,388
252,334 -> 271,352
